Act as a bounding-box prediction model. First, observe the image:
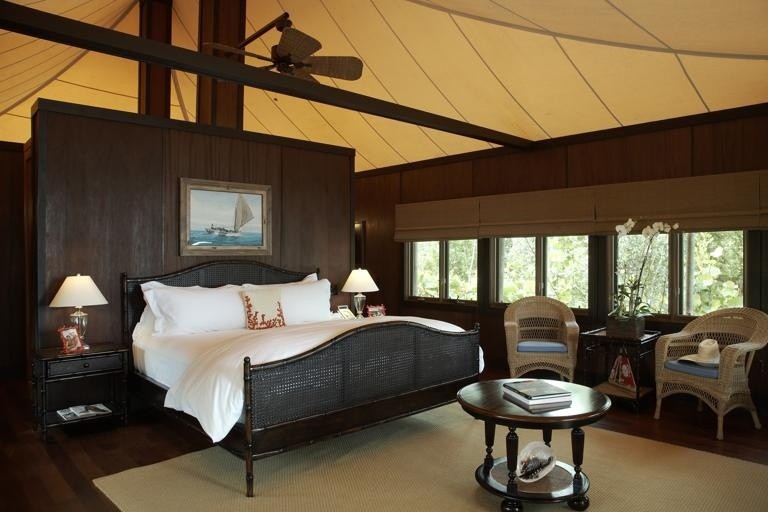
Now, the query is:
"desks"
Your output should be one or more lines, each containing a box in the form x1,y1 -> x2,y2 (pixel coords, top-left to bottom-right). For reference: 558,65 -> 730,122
579,327 -> 661,416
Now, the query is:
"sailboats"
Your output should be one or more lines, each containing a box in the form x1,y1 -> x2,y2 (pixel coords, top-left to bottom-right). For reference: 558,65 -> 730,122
204,192 -> 254,239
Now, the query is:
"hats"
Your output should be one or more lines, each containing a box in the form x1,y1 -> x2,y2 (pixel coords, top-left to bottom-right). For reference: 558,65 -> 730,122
679,339 -> 740,367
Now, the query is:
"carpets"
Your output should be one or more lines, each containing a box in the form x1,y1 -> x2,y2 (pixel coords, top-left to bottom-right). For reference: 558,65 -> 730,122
91,402 -> 766,512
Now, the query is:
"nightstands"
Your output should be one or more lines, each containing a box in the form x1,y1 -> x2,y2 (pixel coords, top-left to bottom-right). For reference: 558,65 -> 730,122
31,342 -> 130,441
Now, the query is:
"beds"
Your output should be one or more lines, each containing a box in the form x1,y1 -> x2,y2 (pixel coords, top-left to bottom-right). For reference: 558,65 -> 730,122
121,260 -> 481,497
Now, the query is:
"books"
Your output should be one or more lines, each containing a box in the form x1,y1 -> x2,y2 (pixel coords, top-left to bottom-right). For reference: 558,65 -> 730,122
503,378 -> 572,399
502,386 -> 572,414
57,408 -> 97,420
70,402 -> 113,416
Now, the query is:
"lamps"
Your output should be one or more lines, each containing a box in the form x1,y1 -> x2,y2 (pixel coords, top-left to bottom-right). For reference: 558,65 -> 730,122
48,273 -> 110,350
341,267 -> 380,321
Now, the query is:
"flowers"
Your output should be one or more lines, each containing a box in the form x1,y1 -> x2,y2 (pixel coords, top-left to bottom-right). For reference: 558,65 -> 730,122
609,217 -> 680,321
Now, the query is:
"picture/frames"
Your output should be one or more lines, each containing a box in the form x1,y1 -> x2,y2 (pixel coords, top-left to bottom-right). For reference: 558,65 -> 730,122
178,176 -> 273,258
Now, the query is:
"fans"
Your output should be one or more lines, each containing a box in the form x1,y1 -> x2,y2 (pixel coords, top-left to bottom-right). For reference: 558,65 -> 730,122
200,27 -> 363,86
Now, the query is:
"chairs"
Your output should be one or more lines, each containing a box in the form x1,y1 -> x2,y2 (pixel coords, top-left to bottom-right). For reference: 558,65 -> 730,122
503,296 -> 580,383
654,306 -> 768,440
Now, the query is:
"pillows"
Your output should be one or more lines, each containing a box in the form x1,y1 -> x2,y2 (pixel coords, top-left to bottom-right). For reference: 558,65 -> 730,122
139,273 -> 333,337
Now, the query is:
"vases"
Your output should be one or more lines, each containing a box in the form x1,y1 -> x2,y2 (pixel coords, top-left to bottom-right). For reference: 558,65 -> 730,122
605,316 -> 646,339
